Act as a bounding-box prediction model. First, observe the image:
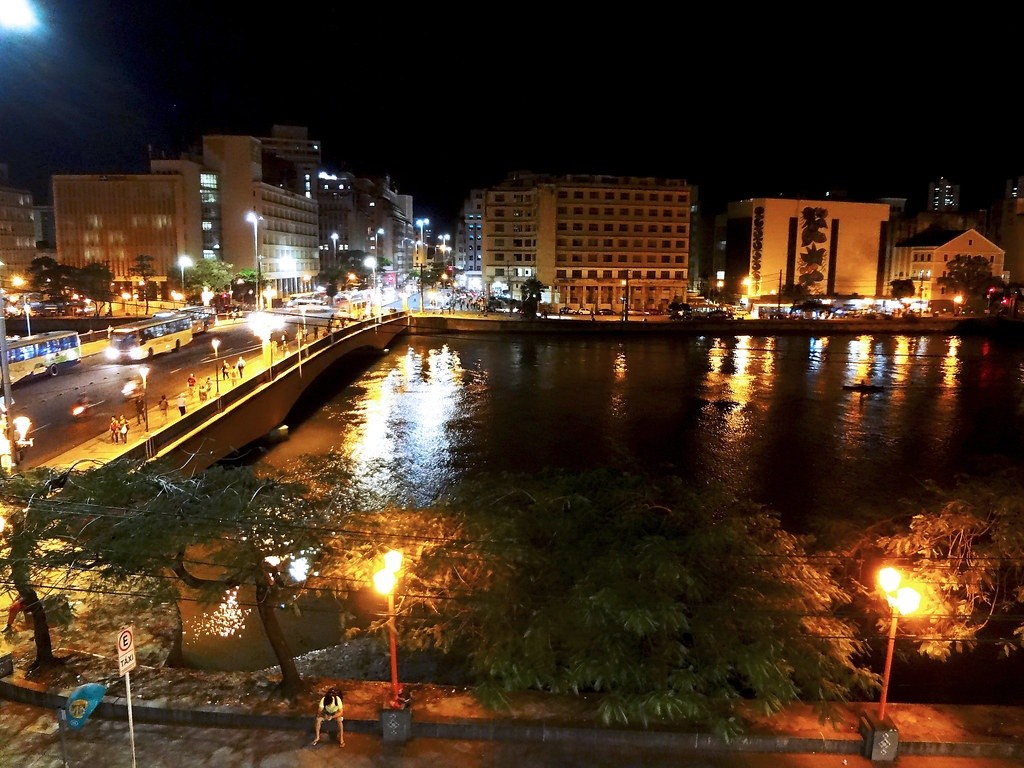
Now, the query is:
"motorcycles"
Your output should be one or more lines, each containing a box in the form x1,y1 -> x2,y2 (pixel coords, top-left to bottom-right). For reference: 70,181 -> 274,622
123,383 -> 145,402
73,401 -> 90,422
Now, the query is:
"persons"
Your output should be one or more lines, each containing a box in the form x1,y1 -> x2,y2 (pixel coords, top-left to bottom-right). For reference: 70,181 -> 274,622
199,376 -> 212,403
236,356 -> 246,378
331,314 -> 349,328
0,594 -> 25,633
283,330 -> 289,357
177,393 -> 188,416
314,324 -> 318,340
312,694 -> 345,748
136,396 -> 146,425
109,414 -> 130,444
158,395 -> 169,417
231,366 -> 238,386
187,373 -> 196,400
221,360 -> 230,381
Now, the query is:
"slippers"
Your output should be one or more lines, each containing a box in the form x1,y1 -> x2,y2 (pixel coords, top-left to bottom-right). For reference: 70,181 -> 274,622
311,739 -> 320,746
339,741 -> 346,748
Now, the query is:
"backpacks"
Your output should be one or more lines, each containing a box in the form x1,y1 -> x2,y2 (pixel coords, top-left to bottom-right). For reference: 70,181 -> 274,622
324,688 -> 343,706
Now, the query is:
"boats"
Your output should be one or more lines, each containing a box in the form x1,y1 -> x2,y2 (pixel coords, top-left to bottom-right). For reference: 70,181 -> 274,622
842,384 -> 884,393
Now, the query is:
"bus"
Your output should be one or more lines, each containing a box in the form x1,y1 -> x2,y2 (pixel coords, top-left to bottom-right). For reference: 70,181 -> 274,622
107,304 -> 217,361
0,330 -> 81,394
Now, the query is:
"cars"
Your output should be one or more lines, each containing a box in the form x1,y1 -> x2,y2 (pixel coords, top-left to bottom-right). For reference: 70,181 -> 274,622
578,308 -> 728,319
558,306 -> 578,315
492,306 -> 506,314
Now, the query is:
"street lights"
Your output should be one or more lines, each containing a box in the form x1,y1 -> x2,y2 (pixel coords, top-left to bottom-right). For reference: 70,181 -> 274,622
140,366 -> 149,432
11,277 -> 31,335
330,233 -> 339,261
373,546 -> 407,708
877,566 -> 915,720
246,212 -> 258,310
437,232 -> 451,261
364,257 -> 376,293
211,338 -> 219,396
415,219 -> 429,310
375,228 -> 385,272
